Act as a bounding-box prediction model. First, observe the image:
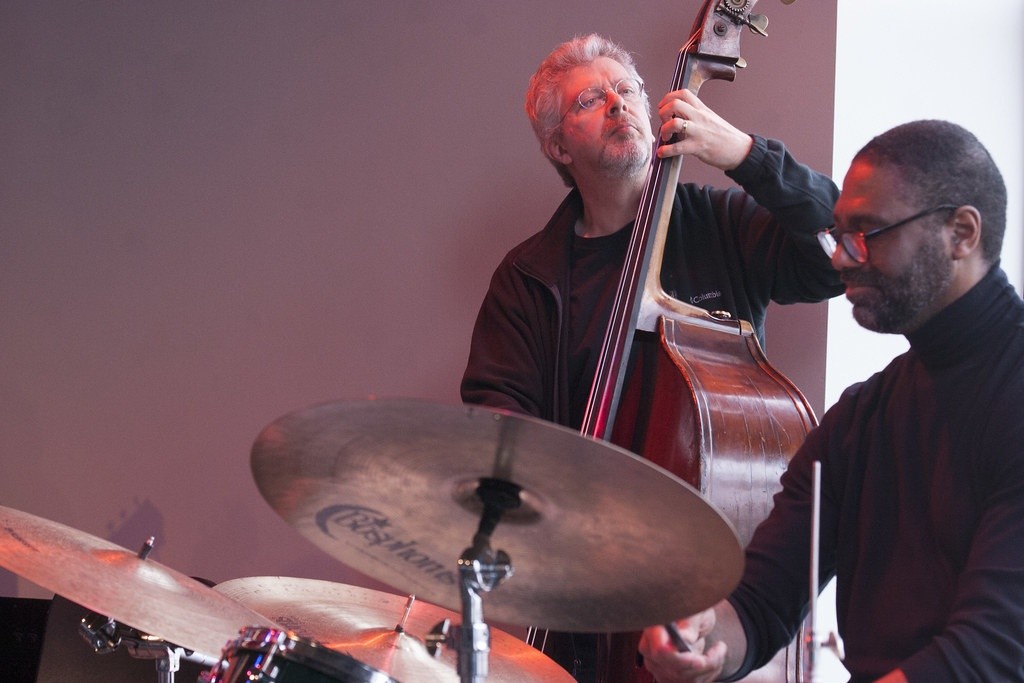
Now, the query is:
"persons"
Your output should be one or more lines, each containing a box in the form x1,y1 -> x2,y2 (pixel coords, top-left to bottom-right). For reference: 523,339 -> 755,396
635,119 -> 1024,683
461,32 -> 848,682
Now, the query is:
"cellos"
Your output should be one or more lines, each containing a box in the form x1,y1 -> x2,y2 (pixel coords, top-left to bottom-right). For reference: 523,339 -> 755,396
524,0 -> 828,682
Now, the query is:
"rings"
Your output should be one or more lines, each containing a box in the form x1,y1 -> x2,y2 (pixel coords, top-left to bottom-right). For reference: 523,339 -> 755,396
681,120 -> 688,134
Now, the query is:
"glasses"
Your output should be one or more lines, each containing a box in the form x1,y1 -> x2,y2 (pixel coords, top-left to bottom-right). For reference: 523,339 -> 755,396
560,77 -> 643,124
813,203 -> 961,264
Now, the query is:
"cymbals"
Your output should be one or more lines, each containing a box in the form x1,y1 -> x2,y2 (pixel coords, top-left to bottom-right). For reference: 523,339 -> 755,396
208,577 -> 580,683
0,506 -> 302,658
249,396 -> 747,633
